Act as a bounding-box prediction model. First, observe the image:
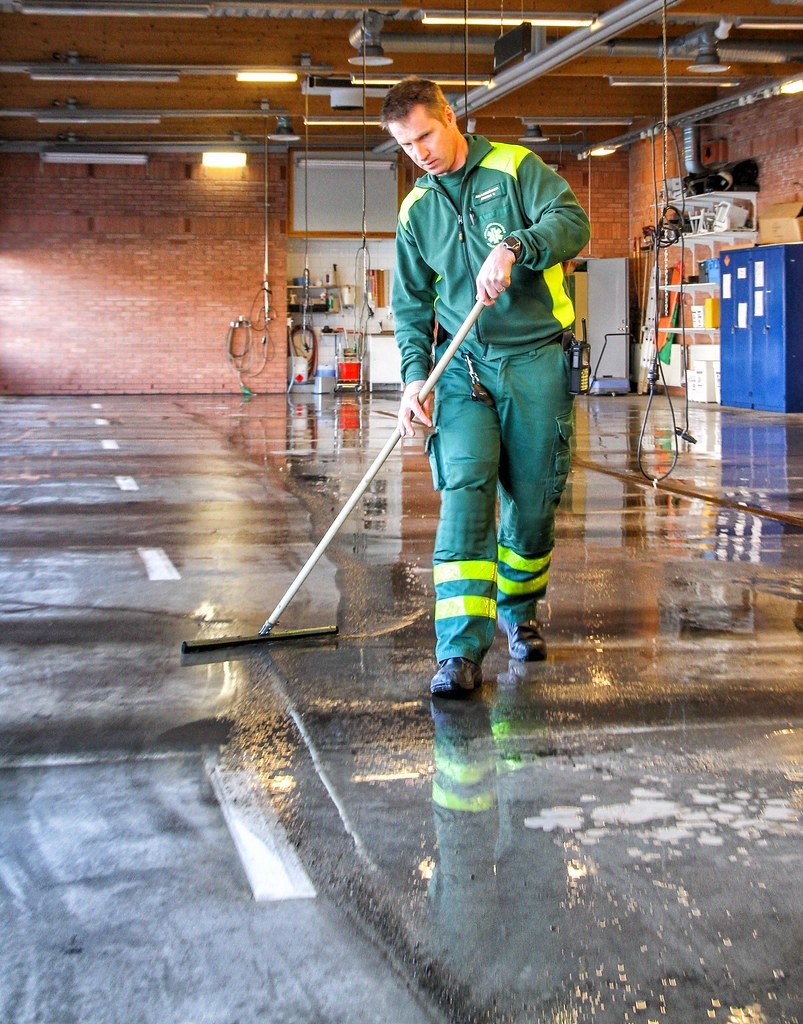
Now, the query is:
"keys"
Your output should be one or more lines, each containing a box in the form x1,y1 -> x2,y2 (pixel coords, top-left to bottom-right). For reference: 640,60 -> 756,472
463,356 -> 495,407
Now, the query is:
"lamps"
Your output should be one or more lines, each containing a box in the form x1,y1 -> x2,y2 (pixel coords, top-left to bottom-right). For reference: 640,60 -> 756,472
421,10 -> 597,27
609,76 -> 743,87
38,116 -> 162,124
29,67 -> 181,82
520,117 -> 635,125
38,150 -> 148,165
303,115 -> 381,125
734,16 -> 803,31
350,73 -> 493,86
19,0 -> 212,19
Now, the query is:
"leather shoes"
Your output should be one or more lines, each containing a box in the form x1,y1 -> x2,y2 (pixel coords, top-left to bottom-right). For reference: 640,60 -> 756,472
430,653 -> 483,697
496,610 -> 548,660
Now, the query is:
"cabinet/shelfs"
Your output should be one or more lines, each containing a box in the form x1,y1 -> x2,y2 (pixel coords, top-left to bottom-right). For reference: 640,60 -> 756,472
719,243 -> 803,413
657,192 -> 758,345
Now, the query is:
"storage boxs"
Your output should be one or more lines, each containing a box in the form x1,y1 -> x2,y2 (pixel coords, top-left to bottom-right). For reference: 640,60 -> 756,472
698,261 -> 709,283
685,345 -> 721,405
708,258 -> 720,283
759,202 -> 803,245
705,298 -> 720,328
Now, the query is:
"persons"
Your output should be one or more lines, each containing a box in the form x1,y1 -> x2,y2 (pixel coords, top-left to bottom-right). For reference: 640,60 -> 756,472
377,77 -> 592,696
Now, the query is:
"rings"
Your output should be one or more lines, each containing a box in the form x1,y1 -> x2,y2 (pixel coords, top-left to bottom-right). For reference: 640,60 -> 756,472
497,287 -> 504,292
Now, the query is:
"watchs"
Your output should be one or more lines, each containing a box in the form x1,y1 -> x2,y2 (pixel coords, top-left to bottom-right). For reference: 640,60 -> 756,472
500,235 -> 523,261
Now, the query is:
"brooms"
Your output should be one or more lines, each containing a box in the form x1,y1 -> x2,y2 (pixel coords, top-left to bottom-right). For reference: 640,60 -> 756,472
178,261 -> 508,669
